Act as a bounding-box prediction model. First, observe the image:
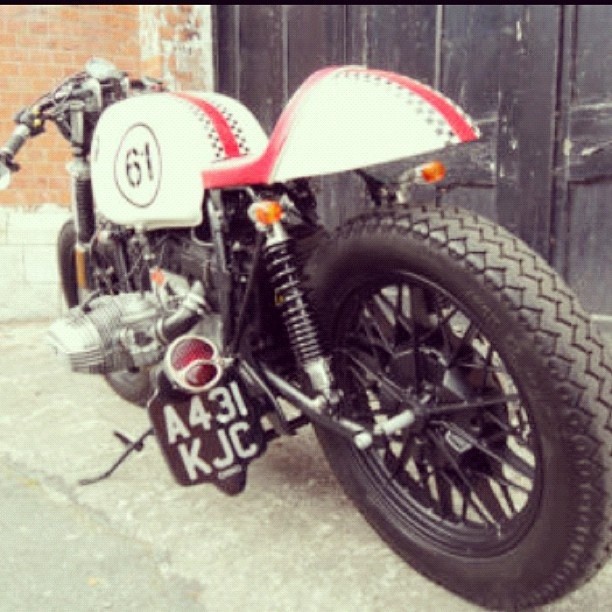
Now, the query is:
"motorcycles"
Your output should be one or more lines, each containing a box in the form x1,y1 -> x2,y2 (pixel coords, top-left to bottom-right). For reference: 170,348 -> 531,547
0,57 -> 612,612
